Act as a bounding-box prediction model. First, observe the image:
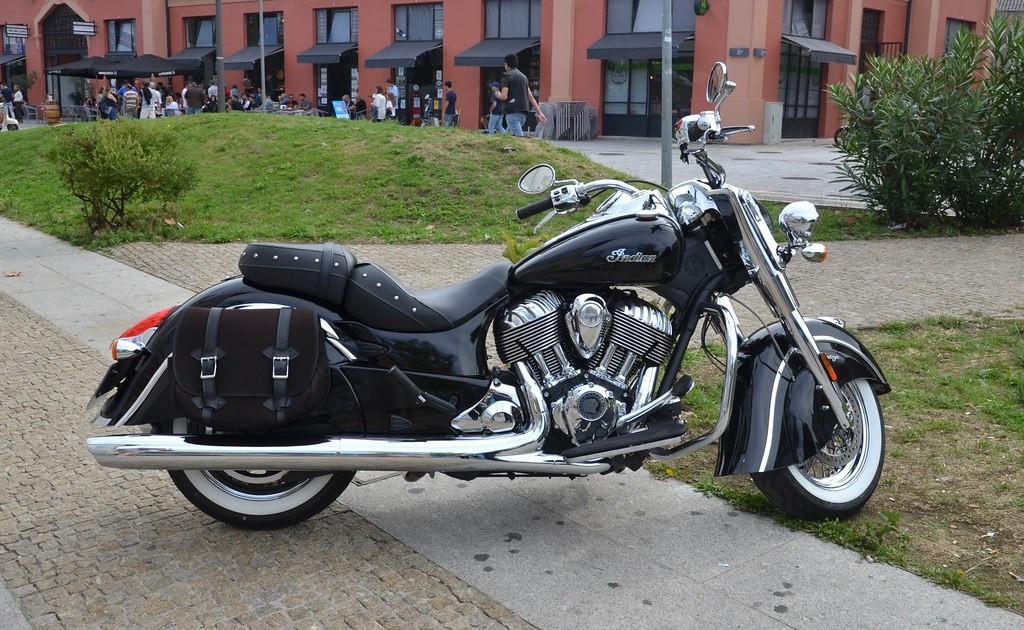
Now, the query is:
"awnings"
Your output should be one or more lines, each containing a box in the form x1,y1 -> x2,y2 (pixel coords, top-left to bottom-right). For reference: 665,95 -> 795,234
224,46 -> 284,70
587,32 -> 694,59
170,47 -> 216,67
296,44 -> 358,65
454,38 -> 540,67
0,54 -> 25,65
781,35 -> 857,65
365,41 -> 443,69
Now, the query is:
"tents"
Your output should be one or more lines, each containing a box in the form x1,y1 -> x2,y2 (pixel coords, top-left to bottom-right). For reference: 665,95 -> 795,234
44,56 -> 113,115
96,53 -> 202,83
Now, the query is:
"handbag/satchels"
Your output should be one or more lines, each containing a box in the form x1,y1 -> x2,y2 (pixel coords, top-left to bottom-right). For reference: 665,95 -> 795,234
99,99 -> 113,115
172,306 -> 329,430
371,106 -> 378,120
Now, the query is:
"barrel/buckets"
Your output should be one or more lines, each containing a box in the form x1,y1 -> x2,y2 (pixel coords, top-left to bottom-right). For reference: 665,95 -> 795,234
45,101 -> 60,125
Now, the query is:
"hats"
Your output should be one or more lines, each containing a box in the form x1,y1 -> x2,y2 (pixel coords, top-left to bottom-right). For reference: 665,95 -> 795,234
490,82 -> 500,88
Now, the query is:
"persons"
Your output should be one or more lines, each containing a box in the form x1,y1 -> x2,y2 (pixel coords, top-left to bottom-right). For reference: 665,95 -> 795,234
495,53 -> 547,135
343,94 -> 366,121
0,83 -> 24,123
370,77 -> 398,122
279,92 -> 312,111
443,81 -> 457,128
488,82 -> 506,135
85,80 -> 274,121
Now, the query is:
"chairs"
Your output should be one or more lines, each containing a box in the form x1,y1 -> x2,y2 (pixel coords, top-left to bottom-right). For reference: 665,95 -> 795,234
60,105 -> 102,122
356,108 -> 370,121
23,104 -> 37,120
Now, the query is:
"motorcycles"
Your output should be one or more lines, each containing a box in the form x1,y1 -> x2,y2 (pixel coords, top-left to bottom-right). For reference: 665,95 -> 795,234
86,61 -> 892,530
